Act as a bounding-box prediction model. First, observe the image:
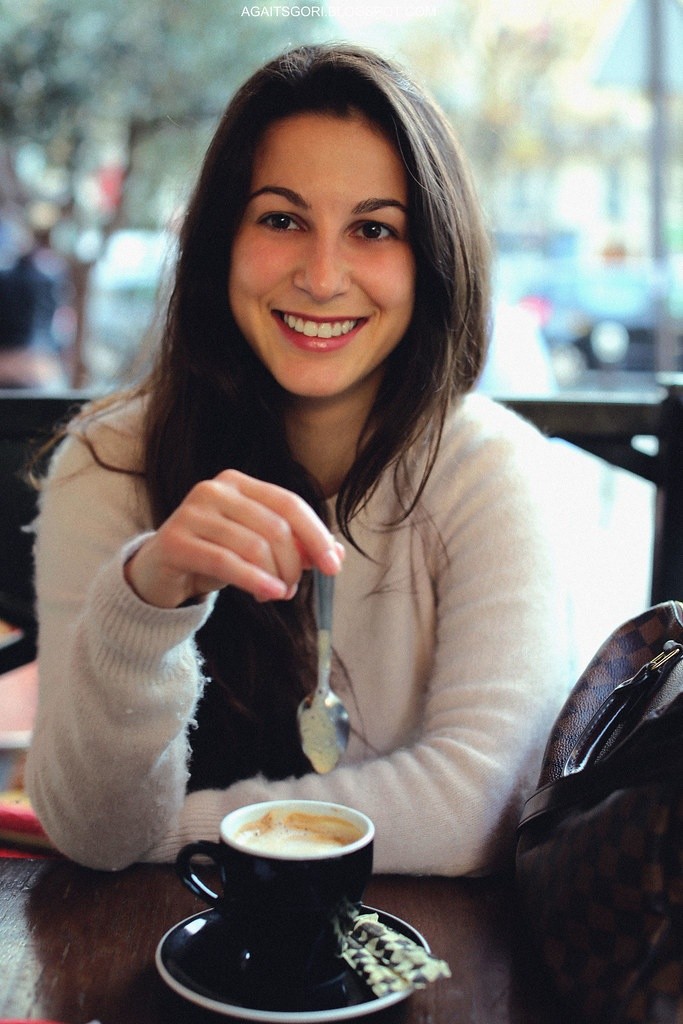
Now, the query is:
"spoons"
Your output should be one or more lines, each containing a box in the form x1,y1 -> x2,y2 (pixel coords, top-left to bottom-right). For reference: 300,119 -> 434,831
297,540 -> 349,776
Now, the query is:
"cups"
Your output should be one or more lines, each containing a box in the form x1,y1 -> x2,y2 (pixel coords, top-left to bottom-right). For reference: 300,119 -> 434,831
176,799 -> 375,982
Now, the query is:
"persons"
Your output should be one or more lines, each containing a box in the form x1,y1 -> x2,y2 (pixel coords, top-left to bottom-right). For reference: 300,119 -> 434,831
0,211 -> 72,392
26,46 -> 591,876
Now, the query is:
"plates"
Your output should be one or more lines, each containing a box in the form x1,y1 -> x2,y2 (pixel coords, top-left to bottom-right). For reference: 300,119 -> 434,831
154,904 -> 431,1024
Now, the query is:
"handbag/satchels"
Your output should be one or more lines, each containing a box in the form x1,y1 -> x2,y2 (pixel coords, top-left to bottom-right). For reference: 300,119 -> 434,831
508,600 -> 683,1024
